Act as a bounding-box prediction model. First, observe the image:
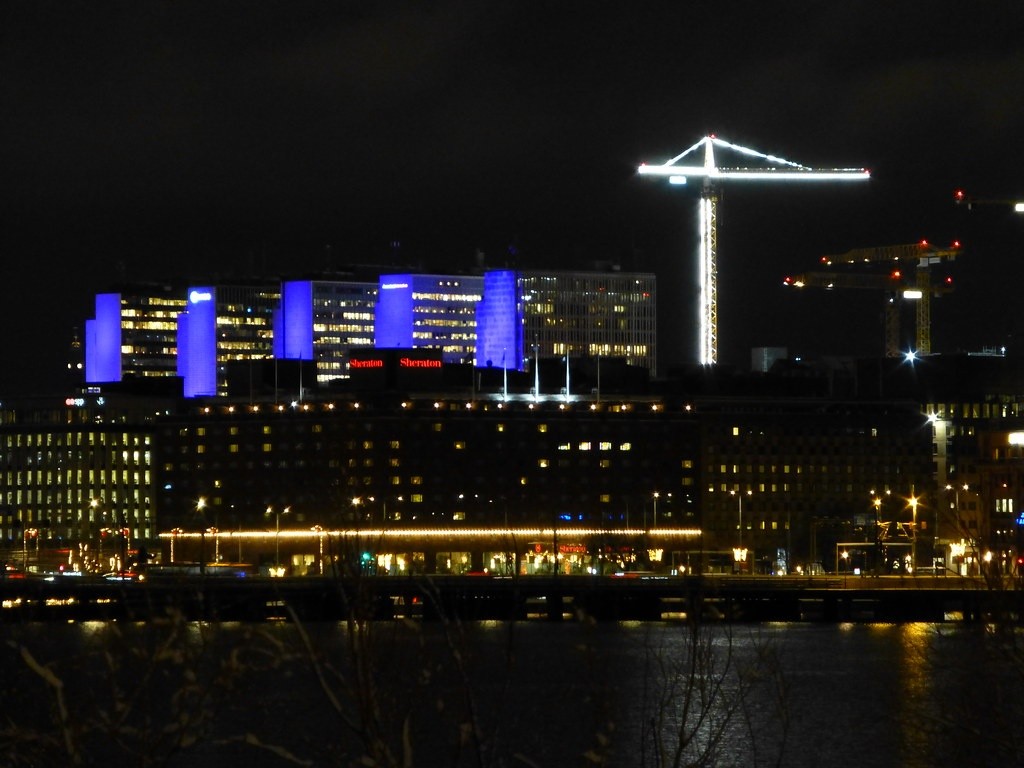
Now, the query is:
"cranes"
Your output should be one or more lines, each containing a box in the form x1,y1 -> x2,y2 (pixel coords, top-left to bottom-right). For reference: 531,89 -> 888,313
953,189 -> 1024,213
636,133 -> 871,364
783,240 -> 960,359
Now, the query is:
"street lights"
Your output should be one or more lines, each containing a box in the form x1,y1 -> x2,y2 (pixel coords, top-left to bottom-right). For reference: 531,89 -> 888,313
653,492 -> 659,528
909,498 -> 918,576
265,505 -> 290,564
731,491 -> 752,546
